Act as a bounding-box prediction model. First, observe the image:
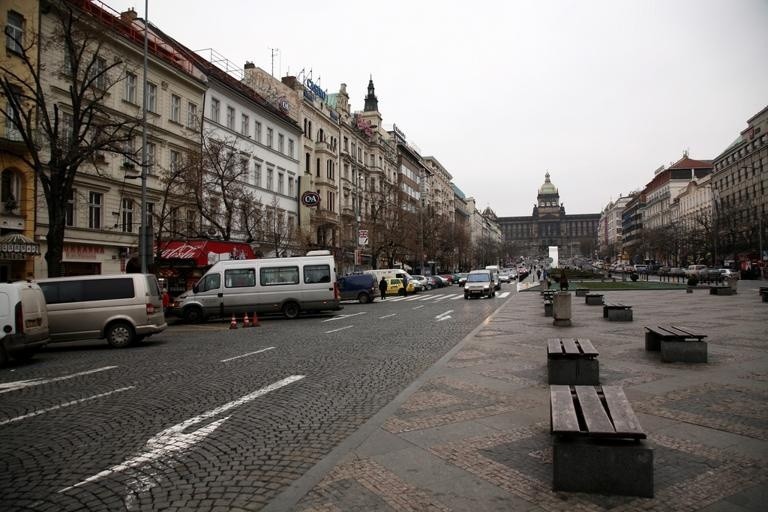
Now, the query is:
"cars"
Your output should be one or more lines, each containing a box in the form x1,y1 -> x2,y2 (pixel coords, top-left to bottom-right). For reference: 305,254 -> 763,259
376,277 -> 414,296
498,265 -> 529,283
492,273 -> 501,290
578,257 -> 739,282
410,271 -> 469,290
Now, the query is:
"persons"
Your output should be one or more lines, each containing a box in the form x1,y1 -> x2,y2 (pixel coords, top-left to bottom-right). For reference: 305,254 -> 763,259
162,288 -> 169,307
380,276 -> 388,300
537,269 -> 541,279
403,274 -> 410,296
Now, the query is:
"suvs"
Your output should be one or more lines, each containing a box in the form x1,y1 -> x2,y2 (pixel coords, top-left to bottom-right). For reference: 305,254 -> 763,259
464,270 -> 495,299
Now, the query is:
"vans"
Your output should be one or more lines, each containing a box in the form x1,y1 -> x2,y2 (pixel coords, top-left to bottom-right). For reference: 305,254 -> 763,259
335,273 -> 382,302
15,273 -> 168,348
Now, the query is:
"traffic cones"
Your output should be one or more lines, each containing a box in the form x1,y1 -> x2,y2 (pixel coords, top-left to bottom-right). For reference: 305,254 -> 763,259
251,310 -> 261,327
240,310 -> 251,327
227,311 -> 240,330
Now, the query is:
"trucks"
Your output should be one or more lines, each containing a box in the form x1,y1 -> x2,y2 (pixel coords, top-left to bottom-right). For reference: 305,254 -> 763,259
0,279 -> 52,358
357,269 -> 422,292
485,265 -> 500,274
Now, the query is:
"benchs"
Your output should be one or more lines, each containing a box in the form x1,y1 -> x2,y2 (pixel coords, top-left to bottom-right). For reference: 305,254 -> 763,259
642,322 -> 710,363
542,287 -> 633,322
548,377 -> 656,495
545,335 -> 599,384
707,285 -> 731,296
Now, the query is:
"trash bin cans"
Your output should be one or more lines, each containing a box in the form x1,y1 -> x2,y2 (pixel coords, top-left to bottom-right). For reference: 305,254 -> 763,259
553,291 -> 572,326
728,272 -> 738,294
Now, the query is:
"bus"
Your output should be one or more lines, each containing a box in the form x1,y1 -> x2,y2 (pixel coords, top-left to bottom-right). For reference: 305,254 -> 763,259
171,248 -> 344,323
